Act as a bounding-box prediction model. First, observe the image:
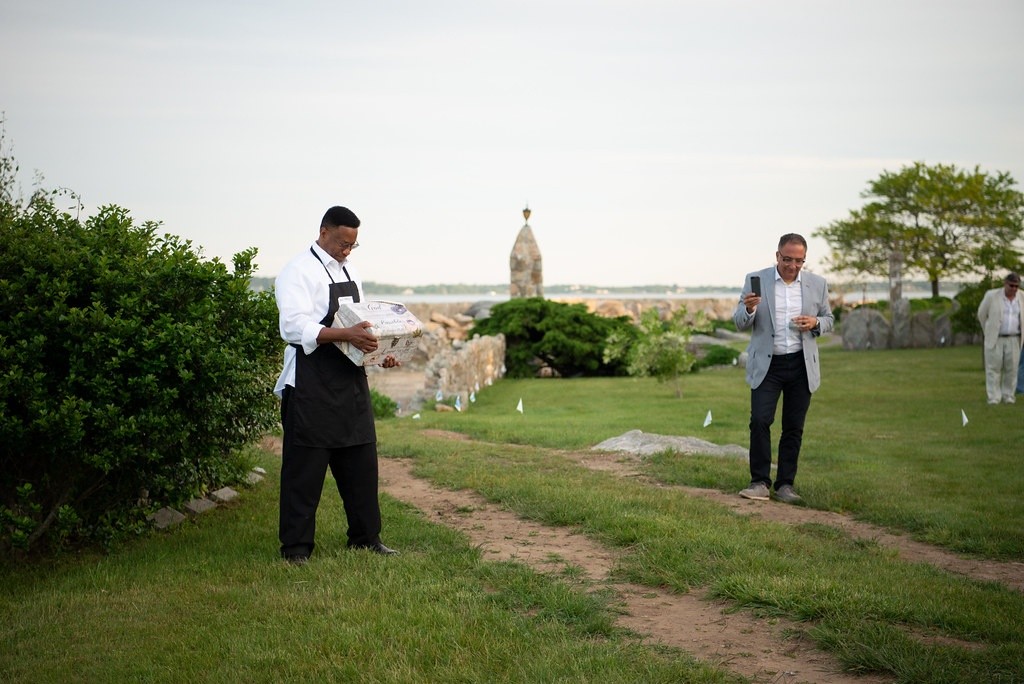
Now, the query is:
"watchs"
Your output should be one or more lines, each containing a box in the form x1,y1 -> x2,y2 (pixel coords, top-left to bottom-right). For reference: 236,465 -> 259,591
815,318 -> 820,330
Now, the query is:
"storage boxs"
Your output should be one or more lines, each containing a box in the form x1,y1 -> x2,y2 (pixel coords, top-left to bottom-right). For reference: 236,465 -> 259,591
329,301 -> 426,366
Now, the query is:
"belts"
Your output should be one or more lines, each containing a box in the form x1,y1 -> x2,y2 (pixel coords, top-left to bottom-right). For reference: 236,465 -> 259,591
998,333 -> 1022,337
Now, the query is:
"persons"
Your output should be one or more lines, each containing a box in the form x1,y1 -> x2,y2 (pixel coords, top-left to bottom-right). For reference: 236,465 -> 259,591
977,273 -> 1024,405
273,206 -> 402,563
734,233 -> 835,503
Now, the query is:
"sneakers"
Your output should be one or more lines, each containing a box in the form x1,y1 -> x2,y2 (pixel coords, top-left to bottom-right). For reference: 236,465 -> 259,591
738,482 -> 772,501
775,485 -> 804,504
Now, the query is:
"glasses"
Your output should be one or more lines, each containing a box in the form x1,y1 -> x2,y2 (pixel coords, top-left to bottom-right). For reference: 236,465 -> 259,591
777,250 -> 806,266
337,242 -> 359,249
1007,281 -> 1020,288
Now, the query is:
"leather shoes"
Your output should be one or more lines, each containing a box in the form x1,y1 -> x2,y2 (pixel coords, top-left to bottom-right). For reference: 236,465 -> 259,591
286,552 -> 310,566
347,539 -> 397,556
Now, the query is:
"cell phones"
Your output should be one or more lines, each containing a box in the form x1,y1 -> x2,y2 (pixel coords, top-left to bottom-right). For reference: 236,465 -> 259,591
750,276 -> 761,298
